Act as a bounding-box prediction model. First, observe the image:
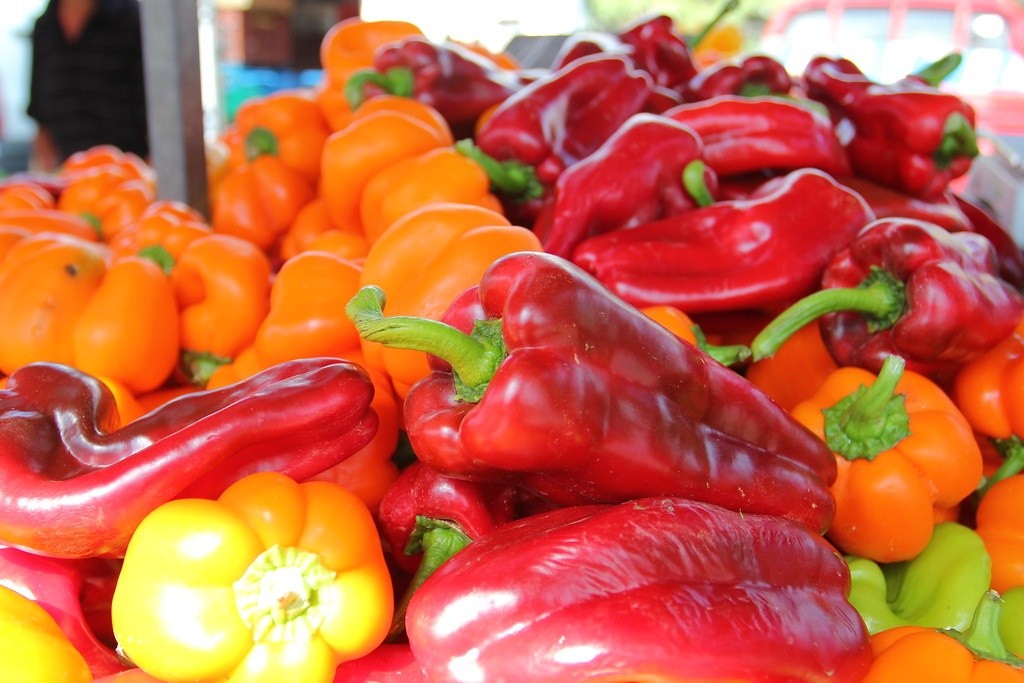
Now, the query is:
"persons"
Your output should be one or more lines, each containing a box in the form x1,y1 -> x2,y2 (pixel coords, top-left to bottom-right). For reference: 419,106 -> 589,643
25,1 -> 155,173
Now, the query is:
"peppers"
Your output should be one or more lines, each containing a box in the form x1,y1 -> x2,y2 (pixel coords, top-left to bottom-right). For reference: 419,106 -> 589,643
0,22 -> 1024,683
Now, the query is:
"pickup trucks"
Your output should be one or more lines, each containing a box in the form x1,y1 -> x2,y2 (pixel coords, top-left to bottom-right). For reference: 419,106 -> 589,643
734,2 -> 1023,248
